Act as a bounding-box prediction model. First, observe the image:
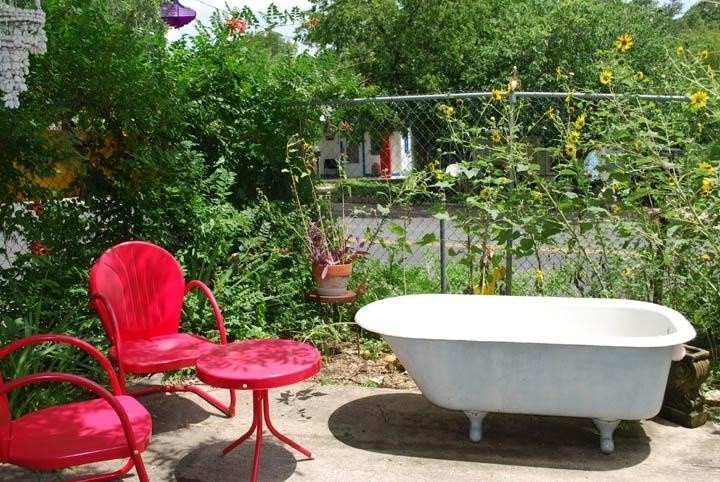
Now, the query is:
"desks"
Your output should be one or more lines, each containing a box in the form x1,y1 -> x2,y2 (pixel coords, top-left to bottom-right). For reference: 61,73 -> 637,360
195,337 -> 322,482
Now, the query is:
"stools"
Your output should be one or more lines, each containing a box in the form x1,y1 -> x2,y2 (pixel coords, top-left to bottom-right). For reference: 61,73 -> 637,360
305,291 -> 360,358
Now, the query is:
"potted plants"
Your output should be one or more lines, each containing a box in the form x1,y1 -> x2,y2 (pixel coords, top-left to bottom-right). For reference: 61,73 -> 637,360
308,221 -> 370,296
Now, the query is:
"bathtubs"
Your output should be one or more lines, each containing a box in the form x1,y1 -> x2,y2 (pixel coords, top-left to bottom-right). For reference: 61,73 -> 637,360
354,294 -> 698,456
